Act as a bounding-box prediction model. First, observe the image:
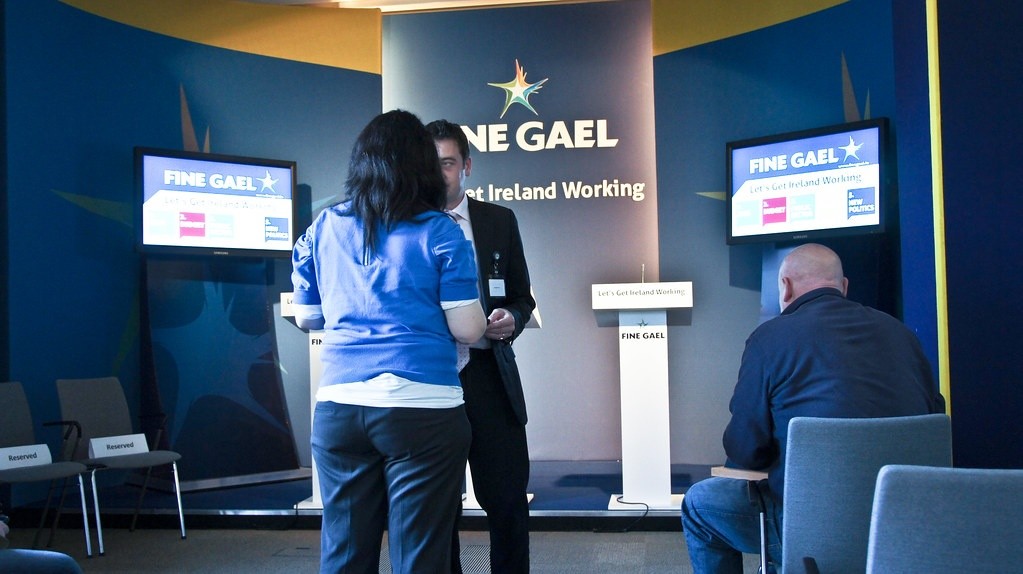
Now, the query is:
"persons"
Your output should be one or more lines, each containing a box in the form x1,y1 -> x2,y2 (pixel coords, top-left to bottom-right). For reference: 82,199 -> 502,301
420,118 -> 536,574
681,243 -> 946,574
287,108 -> 489,573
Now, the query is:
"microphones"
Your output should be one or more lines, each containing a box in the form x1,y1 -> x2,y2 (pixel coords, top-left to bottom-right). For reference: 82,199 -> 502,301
641,264 -> 644,283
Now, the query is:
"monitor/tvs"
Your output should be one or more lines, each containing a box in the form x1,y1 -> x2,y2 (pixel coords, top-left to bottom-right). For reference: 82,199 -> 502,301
725,117 -> 891,244
132,145 -> 297,257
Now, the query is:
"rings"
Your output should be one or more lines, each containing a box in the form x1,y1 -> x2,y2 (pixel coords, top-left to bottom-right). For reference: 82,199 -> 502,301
499,334 -> 505,340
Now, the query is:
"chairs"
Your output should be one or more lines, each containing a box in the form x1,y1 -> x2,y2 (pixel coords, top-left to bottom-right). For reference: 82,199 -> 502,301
0,380 -> 74,549
712,413 -> 953,573
56,374 -> 188,555
803,464 -> 1023,574
